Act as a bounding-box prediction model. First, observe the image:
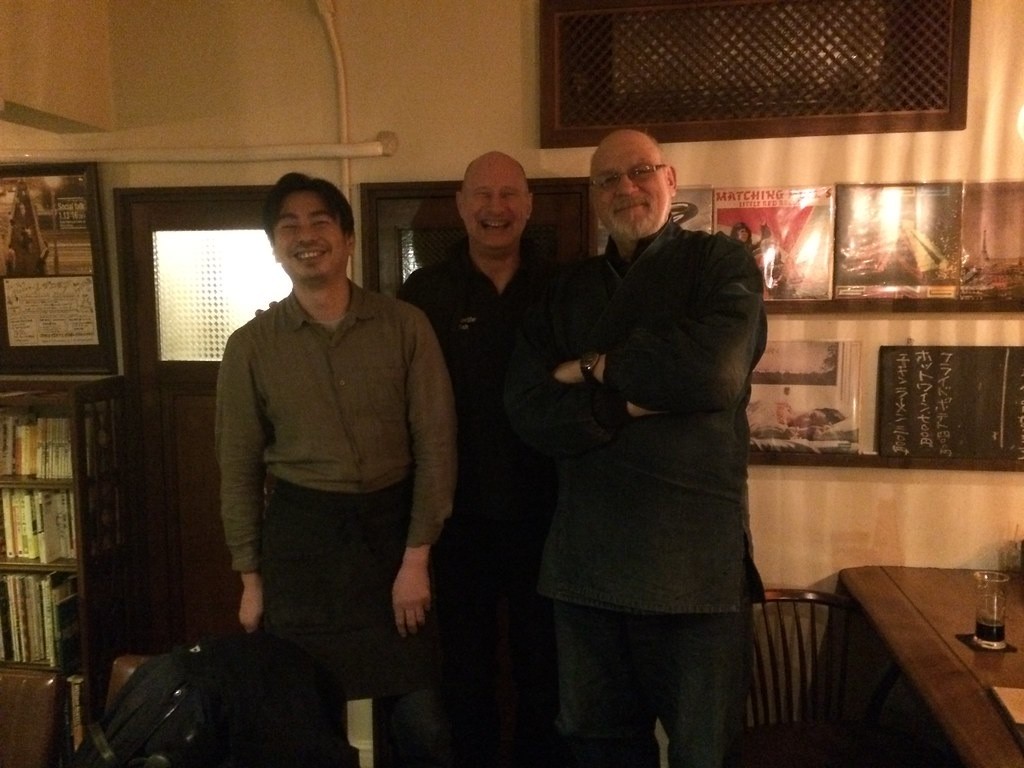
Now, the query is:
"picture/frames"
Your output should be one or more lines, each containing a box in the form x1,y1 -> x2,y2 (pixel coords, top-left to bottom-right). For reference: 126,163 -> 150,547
0,160 -> 116,375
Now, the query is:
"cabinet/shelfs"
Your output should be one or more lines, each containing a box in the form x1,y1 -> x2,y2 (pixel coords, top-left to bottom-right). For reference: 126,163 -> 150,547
0,376 -> 130,707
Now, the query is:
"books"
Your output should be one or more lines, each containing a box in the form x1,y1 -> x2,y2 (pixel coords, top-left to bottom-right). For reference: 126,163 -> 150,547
1,488 -> 77,563
0,405 -> 96,479
0,571 -> 79,667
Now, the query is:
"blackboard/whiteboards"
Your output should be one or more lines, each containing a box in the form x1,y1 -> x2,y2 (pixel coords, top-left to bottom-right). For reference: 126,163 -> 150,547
878,346 -> 1024,460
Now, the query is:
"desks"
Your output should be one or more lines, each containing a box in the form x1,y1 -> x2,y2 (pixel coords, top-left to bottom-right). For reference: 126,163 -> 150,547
839,566 -> 1024,768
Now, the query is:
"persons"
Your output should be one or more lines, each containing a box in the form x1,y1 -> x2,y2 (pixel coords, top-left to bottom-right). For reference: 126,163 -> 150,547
747,400 -> 844,440
216,173 -> 461,767
502,127 -> 766,768
396,153 -> 569,768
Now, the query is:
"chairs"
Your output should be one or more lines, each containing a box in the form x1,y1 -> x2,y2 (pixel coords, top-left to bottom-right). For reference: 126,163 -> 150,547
745,590 -> 951,767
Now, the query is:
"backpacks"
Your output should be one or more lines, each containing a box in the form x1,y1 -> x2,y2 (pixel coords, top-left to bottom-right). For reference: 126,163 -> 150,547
66,651 -> 221,768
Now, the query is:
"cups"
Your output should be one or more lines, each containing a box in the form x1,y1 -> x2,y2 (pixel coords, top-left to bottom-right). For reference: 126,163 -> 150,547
974,571 -> 1009,649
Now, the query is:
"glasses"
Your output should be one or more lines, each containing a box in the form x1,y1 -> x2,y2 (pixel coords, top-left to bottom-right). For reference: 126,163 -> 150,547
591,165 -> 666,190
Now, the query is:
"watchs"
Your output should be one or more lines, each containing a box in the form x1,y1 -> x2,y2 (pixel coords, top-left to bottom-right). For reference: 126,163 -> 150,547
580,352 -> 599,383
